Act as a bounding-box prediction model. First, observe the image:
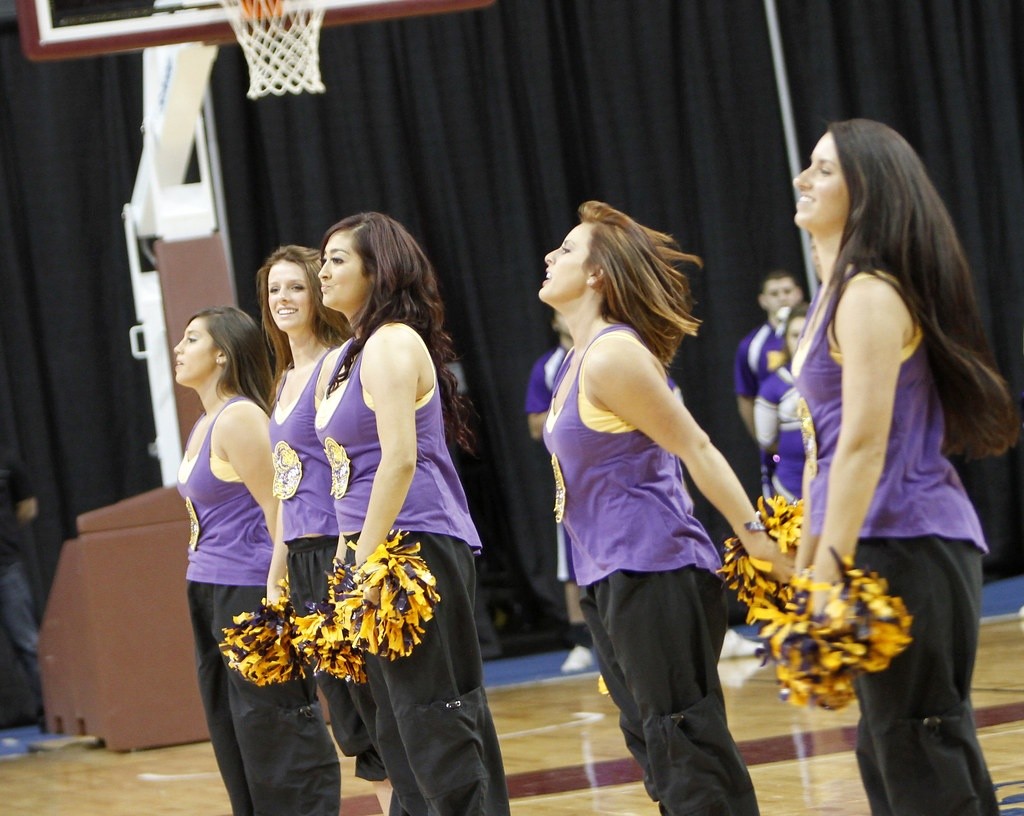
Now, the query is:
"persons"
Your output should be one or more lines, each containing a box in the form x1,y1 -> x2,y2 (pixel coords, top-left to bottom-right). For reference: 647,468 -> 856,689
539,201 -> 797,816
257,245 -> 395,816
314,212 -> 511,816
735,270 -> 816,510
525,310 -> 595,673
173,307 -> 341,816
0,457 -> 54,733
792,120 -> 1016,816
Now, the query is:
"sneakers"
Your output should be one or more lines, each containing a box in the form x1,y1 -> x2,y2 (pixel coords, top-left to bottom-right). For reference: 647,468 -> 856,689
560,643 -> 594,671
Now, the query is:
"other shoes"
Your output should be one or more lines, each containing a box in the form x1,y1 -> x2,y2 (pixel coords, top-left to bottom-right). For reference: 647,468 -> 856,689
718,629 -> 762,659
